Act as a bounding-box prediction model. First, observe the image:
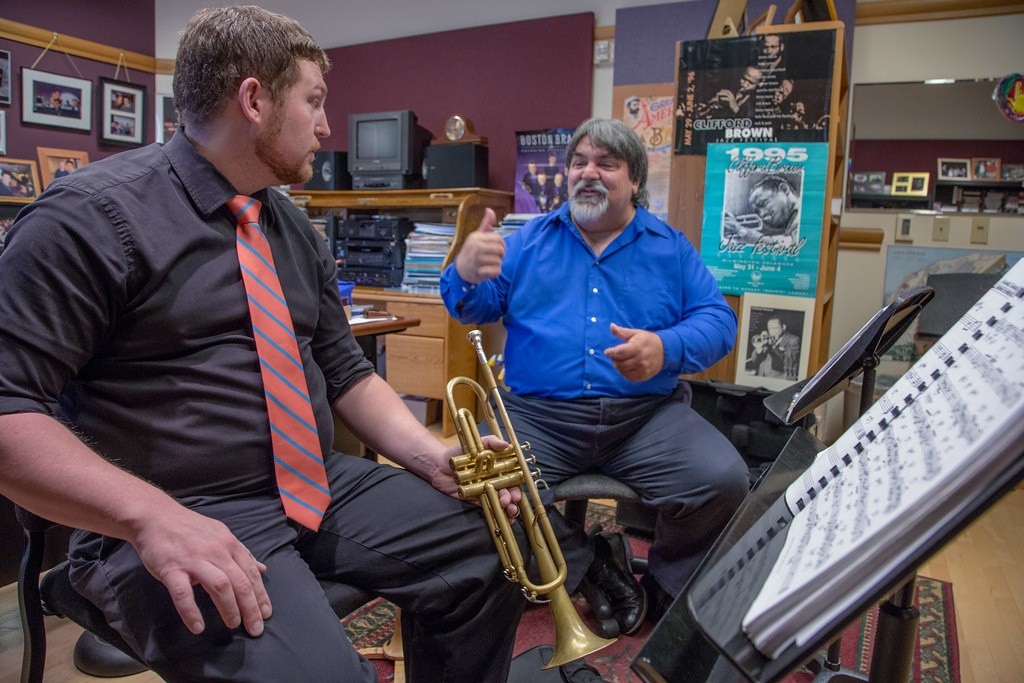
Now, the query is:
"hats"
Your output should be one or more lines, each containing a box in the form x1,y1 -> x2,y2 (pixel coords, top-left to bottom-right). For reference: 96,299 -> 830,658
625,94 -> 639,106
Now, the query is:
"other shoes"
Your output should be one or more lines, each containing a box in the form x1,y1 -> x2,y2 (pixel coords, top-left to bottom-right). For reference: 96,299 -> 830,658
639,575 -> 673,624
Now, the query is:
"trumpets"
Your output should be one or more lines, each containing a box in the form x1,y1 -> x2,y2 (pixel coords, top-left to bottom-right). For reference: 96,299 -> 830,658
724,211 -> 773,238
441,325 -> 620,669
693,88 -> 748,117
750,335 -> 777,349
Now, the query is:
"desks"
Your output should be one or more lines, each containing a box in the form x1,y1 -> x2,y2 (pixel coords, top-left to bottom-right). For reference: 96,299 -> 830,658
333,311 -> 421,466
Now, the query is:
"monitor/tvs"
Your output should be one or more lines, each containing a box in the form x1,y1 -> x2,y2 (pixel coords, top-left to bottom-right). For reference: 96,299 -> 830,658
347,110 -> 436,189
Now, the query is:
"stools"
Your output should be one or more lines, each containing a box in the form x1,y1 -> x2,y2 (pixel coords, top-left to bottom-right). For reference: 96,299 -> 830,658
531,472 -> 651,637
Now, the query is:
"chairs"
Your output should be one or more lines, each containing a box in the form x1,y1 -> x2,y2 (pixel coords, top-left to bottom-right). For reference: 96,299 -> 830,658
17,506 -> 377,683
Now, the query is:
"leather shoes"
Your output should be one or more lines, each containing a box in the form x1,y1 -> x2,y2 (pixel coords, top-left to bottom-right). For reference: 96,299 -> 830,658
594,530 -> 647,636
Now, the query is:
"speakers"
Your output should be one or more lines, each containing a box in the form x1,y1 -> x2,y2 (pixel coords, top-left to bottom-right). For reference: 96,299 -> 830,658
304,150 -> 352,190
421,144 -> 489,188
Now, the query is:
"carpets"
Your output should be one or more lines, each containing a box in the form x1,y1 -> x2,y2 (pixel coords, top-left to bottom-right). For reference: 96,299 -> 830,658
341,526 -> 959,683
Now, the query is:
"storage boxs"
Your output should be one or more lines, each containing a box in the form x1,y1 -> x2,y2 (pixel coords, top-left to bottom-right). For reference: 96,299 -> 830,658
400,395 -> 439,426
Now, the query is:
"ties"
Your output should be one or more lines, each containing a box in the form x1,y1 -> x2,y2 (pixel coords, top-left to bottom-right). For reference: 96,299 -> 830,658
230,196 -> 331,533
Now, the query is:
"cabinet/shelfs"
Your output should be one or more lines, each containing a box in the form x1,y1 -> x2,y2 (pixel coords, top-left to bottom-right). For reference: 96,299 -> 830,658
288,187 -> 513,438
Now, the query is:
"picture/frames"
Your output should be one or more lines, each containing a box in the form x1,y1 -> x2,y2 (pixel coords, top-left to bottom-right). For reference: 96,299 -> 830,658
852,171 -> 886,194
0,157 -> 41,204
892,172 -> 929,197
937,158 -> 971,181
0,49 -> 12,105
972,158 -> 1001,182
20,67 -> 92,134
98,76 -> 148,148
37,147 -> 90,189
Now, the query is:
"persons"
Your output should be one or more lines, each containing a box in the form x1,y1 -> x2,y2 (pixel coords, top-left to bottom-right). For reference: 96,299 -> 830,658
750,316 -> 800,381
710,35 -> 805,130
439,118 -> 749,634
523,152 -> 566,213
0,5 -> 529,682
0,89 -> 135,197
724,175 -> 800,248
